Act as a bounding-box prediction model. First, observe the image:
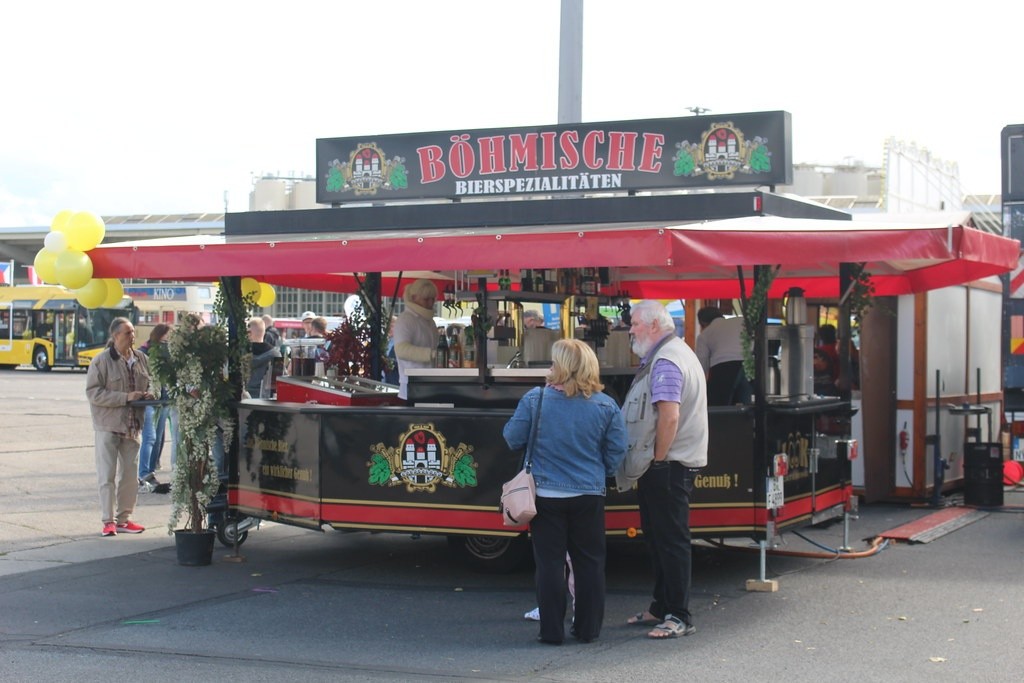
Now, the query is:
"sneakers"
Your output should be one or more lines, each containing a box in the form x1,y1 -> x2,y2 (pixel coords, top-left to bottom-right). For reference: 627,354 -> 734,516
523,605 -> 540,622
138,474 -> 161,489
113,519 -> 144,535
102,521 -> 117,538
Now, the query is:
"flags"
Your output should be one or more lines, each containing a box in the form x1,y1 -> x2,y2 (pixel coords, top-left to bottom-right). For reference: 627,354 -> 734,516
27,266 -> 44,285
0,262 -> 11,285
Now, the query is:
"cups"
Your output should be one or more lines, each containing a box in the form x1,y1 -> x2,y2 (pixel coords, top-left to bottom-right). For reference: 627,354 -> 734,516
314,362 -> 326,378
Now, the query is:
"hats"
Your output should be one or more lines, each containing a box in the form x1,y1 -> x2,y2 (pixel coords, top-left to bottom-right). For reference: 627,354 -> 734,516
303,311 -> 317,323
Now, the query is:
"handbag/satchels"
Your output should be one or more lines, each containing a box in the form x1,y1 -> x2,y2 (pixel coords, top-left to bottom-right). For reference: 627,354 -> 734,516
496,467 -> 538,532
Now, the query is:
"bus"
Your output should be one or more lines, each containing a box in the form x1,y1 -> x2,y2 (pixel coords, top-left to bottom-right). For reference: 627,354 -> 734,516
0,282 -> 140,370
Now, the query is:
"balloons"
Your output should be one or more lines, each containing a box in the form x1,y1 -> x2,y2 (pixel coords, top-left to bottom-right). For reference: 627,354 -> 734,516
100,278 -> 124,309
239,277 -> 262,303
33,210 -> 106,291
76,278 -> 108,309
256,281 -> 276,308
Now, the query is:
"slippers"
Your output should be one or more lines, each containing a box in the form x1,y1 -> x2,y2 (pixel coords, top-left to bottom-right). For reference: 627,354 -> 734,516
628,605 -> 666,625
648,614 -> 698,640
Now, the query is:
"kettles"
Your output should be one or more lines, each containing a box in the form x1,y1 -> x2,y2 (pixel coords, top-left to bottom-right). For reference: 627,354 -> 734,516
781,286 -> 807,326
769,355 -> 781,395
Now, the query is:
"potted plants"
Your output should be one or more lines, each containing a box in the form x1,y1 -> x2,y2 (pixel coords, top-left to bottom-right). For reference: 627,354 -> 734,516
147,319 -> 236,566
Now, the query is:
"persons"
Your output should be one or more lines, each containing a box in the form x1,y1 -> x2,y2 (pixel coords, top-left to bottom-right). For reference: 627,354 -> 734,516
243,317 -> 286,400
393,278 -> 442,402
137,323 -> 176,495
85,316 -> 157,538
164,312 -> 208,473
693,305 -> 754,406
287,310 -> 400,387
197,323 -> 251,536
261,314 -> 283,400
522,308 -> 544,329
813,323 -> 843,401
500,339 -> 630,647
614,299 -> 708,642
834,336 -> 861,391
811,346 -> 844,397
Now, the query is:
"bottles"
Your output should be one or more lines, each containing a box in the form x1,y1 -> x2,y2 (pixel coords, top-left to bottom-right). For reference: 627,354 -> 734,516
463,334 -> 474,368
436,325 -> 448,367
448,327 -> 462,367
499,269 -> 602,295
